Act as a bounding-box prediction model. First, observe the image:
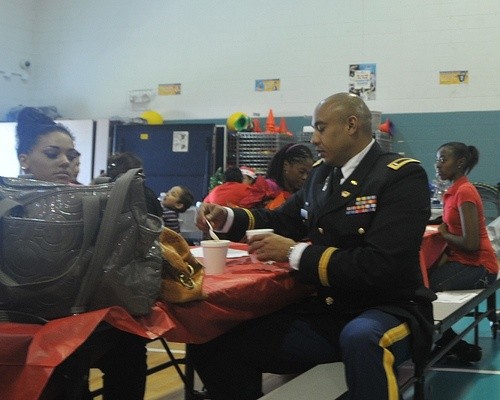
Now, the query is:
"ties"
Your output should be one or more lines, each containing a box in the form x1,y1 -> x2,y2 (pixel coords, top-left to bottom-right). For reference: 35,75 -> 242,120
316,167 -> 343,203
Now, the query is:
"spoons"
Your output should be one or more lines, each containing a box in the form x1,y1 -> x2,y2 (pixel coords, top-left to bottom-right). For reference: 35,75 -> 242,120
203,216 -> 220,241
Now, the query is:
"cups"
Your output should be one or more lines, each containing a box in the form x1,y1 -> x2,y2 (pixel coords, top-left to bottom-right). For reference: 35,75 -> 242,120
246,229 -> 275,263
200,239 -> 231,276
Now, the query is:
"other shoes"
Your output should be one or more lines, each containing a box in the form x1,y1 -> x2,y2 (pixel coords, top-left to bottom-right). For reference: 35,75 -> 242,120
430,343 -> 482,364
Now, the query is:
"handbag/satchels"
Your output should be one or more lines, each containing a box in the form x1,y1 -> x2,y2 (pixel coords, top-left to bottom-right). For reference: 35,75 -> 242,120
0,168 -> 164,325
158,225 -> 205,303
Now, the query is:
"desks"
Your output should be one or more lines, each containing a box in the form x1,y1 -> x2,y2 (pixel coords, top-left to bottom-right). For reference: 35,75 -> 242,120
0,224 -> 448,400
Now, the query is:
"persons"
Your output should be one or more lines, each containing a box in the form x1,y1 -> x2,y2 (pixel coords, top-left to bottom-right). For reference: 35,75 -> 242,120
162,186 -> 193,231
428,142 -> 500,368
14,106 -> 148,400
239,165 -> 256,185
201,168 -> 261,207
104,152 -> 163,218
244,144 -> 316,212
185,91 -> 437,400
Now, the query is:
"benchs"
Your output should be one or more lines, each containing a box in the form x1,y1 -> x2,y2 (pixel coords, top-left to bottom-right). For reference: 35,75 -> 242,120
257,272 -> 500,400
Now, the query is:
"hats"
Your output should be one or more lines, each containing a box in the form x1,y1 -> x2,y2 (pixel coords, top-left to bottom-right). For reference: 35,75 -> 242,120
240,166 -> 256,178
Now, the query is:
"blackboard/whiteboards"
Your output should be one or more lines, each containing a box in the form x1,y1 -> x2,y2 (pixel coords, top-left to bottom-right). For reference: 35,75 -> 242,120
114,125 -> 213,206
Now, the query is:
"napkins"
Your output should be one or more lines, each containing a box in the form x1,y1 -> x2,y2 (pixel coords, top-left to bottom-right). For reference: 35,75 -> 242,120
188,247 -> 250,258
